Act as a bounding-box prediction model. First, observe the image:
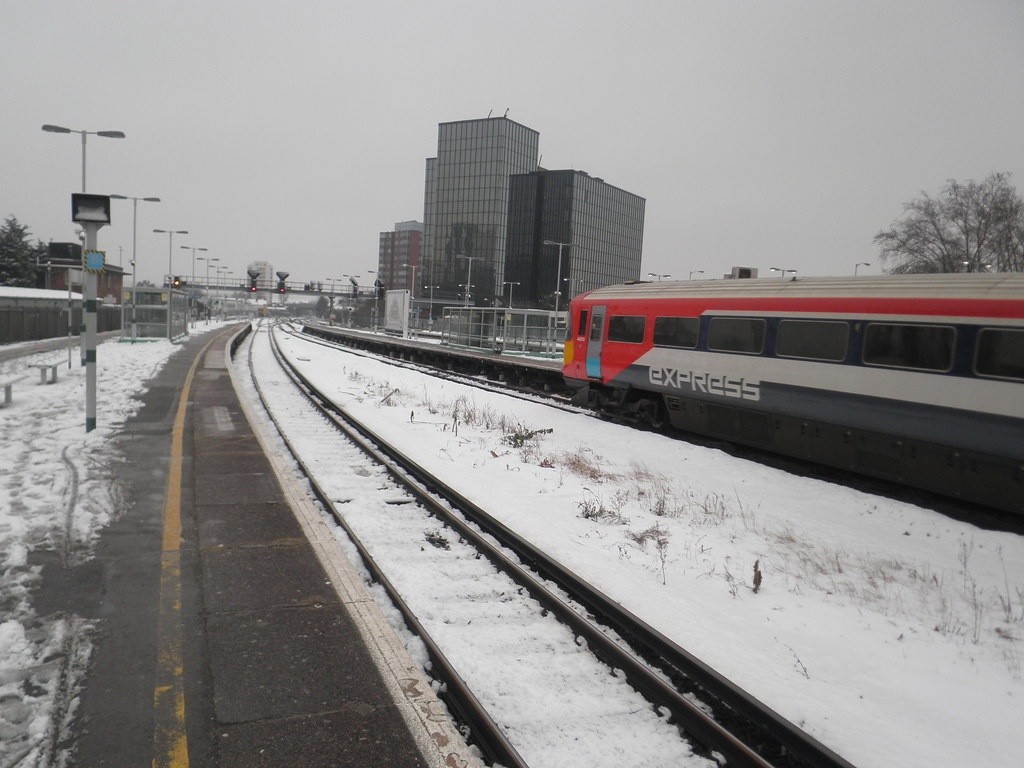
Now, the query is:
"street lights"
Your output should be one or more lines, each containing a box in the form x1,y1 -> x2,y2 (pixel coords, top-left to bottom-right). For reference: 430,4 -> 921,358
769,267 -> 798,278
564,278 -> 584,301
153,229 -> 189,340
40,124 -> 126,366
367,270 -> 387,334
855,262 -> 871,276
424,285 -> 439,332
208,263 -> 234,324
647,273 -> 672,282
326,277 -> 342,327
109,195 -> 161,345
196,257 -> 220,326
403,264 -> 424,339
456,255 -> 486,307
543,240 -> 579,359
502,281 -> 520,342
689,270 -> 705,280
342,274 -> 361,329
180,245 -> 208,329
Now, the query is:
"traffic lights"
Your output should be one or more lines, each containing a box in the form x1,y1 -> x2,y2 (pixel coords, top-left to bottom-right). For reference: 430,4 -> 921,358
279,282 -> 286,294
174,276 -> 181,288
251,280 -> 257,292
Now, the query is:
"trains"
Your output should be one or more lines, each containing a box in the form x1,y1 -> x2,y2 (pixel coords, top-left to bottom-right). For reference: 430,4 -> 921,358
560,272 -> 1024,521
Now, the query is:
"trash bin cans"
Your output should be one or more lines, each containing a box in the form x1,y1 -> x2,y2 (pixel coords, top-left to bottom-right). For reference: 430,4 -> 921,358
494,346 -> 501,355
